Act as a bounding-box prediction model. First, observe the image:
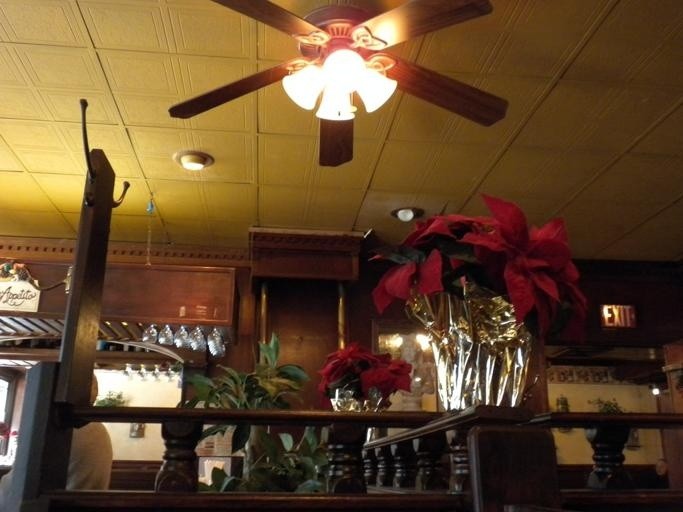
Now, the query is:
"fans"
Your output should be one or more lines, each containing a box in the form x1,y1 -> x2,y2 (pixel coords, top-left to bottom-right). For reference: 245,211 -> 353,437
166,0 -> 510,171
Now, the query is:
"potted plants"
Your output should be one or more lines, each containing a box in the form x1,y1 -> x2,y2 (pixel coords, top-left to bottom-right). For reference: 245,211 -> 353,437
581,394 -> 641,490
176,327 -> 313,493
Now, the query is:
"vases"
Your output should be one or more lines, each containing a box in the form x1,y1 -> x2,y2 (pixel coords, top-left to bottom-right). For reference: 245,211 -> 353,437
415,291 -> 533,410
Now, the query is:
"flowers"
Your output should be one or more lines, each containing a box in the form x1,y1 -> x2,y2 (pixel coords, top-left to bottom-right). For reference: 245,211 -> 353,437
313,337 -> 415,411
363,188 -> 594,354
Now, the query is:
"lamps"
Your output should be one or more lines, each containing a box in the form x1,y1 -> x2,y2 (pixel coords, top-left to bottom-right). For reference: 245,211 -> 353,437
172,149 -> 215,171
280,37 -> 400,123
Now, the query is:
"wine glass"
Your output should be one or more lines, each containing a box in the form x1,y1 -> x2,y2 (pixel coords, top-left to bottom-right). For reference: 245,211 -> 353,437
123,364 -> 182,384
139,324 -> 225,358
